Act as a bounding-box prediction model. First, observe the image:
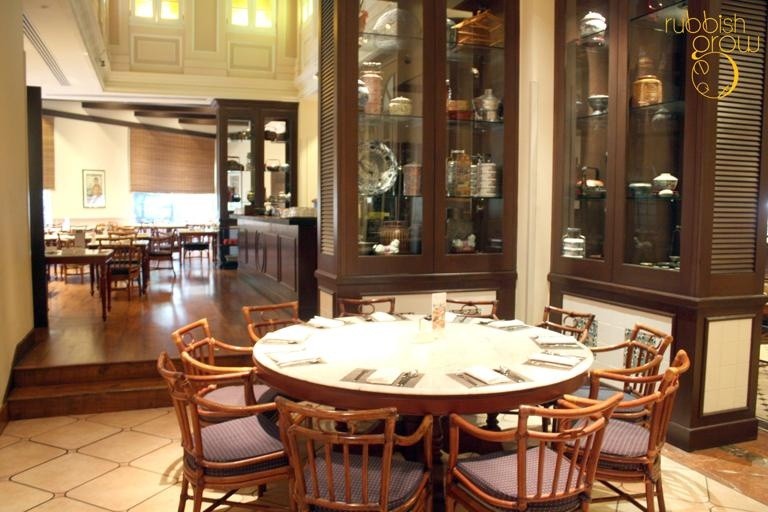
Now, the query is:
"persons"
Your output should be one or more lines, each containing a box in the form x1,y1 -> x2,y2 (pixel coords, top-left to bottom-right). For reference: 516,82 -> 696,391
91,177 -> 102,198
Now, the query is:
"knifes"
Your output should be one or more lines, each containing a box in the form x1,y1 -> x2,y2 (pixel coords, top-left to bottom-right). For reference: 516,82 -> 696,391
492,368 -> 522,384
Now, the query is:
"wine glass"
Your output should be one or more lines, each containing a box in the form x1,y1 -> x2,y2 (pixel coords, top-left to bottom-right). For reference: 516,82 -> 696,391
585,95 -> 608,116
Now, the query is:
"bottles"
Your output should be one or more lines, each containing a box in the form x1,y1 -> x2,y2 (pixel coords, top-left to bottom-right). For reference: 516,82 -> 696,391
631,76 -> 663,109
444,18 -> 458,49
354,207 -> 475,254
560,228 -> 587,259
357,61 -> 503,123
579,10 -> 606,38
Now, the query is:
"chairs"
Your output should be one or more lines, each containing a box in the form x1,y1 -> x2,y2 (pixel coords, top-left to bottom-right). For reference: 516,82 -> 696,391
43,224 -> 221,304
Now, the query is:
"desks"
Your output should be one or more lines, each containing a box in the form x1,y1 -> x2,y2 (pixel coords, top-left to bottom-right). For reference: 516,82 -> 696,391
44,249 -> 116,322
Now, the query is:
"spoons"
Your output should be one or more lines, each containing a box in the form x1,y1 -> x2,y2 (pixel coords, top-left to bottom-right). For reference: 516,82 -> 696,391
400,369 -> 419,386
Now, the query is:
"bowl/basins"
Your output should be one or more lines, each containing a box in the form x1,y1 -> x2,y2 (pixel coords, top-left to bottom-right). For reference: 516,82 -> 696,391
652,172 -> 678,191
226,131 -> 290,202
627,184 -> 652,197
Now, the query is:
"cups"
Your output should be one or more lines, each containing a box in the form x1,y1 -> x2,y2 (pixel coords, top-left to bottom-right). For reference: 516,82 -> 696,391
638,255 -> 680,270
393,157 -> 497,198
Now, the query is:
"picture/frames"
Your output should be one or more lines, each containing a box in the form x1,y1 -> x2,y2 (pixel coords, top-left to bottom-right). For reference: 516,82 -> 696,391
83,169 -> 106,208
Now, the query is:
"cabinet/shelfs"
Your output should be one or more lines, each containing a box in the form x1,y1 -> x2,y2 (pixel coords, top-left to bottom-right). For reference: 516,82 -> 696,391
316,0 -> 519,318
209,97 -> 299,270
545,0 -> 768,453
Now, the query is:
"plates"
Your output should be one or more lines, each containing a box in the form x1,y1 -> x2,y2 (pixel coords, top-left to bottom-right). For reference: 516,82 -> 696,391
358,141 -> 400,196
